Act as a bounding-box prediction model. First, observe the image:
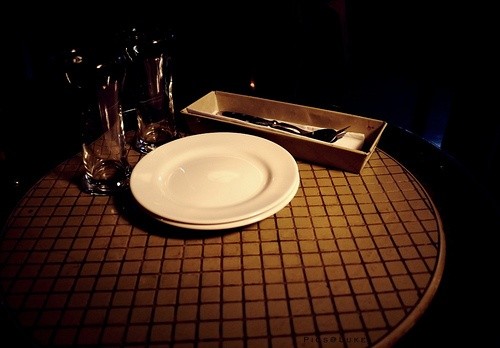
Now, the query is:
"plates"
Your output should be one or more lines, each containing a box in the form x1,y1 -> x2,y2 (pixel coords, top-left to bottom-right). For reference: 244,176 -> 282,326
130,132 -> 301,230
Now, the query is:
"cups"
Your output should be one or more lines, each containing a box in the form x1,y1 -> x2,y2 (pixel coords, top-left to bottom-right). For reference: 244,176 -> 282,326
71,49 -> 132,197
122,23 -> 182,155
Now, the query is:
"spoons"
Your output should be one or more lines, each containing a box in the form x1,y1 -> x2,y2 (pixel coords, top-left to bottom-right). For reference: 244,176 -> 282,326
218,108 -> 352,146
